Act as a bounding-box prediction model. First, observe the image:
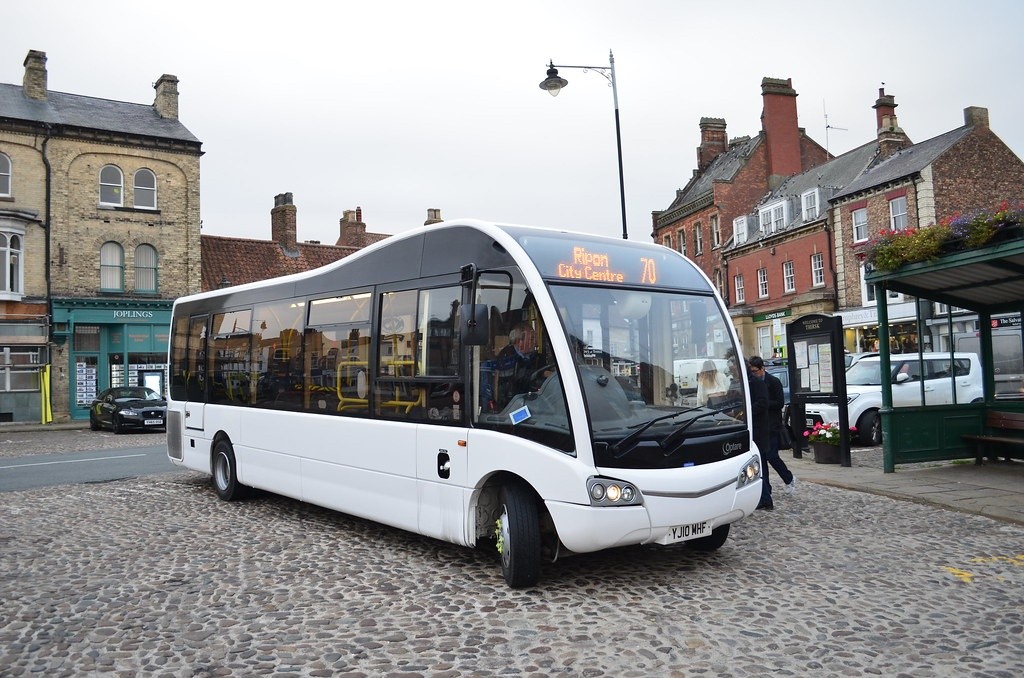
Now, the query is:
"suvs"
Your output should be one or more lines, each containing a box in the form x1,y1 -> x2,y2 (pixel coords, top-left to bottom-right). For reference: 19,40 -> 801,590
805,350 -> 985,448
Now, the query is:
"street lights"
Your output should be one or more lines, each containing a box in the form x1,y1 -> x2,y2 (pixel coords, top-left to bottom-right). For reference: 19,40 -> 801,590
535,45 -> 639,386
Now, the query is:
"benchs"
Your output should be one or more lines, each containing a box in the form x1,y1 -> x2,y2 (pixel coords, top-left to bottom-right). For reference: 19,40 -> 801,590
959,411 -> 1024,466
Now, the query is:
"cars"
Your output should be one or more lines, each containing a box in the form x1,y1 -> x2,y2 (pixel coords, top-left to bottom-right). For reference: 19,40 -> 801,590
843,351 -> 898,371
88,385 -> 167,435
671,357 -> 789,426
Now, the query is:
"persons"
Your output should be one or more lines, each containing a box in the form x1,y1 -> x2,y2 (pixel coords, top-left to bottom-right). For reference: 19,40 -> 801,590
696,347 -> 796,511
491,323 -> 554,412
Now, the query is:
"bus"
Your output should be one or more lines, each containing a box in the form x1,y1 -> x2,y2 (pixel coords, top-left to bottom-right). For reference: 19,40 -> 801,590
164,218 -> 764,590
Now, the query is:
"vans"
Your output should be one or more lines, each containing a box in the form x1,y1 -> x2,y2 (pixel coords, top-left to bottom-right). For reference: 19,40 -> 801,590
953,326 -> 1024,401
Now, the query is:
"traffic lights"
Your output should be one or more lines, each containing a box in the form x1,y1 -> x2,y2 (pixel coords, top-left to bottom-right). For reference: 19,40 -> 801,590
261,322 -> 267,329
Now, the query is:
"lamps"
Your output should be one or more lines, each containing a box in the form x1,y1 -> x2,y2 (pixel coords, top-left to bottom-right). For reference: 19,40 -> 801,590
824,223 -> 829,228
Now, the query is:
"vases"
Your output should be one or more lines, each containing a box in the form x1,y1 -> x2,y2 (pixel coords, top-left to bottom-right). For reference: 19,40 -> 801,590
813,442 -> 842,464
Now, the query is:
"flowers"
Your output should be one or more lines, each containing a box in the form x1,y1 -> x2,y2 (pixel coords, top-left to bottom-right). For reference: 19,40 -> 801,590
864,198 -> 1024,273
802,420 -> 856,446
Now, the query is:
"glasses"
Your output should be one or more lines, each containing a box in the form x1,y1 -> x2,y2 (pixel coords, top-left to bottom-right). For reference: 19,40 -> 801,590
752,369 -> 760,373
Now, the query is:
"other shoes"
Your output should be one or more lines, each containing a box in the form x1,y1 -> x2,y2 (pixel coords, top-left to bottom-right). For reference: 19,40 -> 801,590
756,500 -> 773,509
783,475 -> 796,495
